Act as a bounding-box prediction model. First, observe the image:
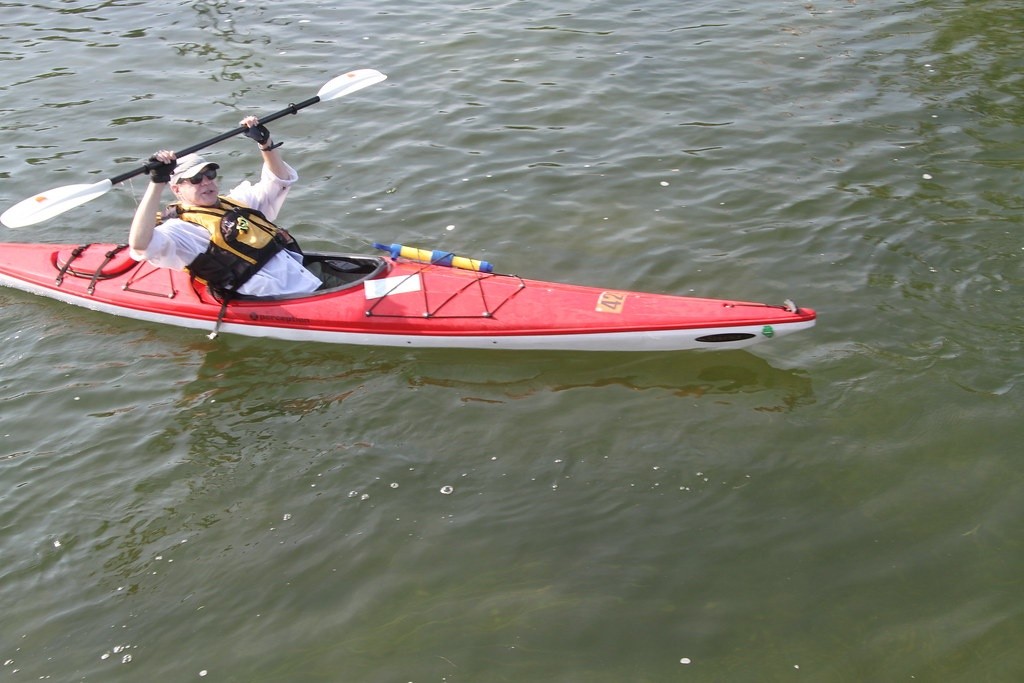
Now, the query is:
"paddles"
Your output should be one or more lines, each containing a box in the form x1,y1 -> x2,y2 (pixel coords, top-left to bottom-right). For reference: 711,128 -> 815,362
1,67 -> 388,228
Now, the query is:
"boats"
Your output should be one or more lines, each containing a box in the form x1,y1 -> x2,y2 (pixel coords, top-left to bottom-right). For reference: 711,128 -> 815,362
0,240 -> 817,357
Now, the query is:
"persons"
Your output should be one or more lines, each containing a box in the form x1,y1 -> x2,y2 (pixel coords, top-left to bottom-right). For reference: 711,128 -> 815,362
129,116 -> 364,298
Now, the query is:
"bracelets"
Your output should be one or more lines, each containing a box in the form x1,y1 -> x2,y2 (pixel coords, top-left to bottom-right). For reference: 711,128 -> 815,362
257,139 -> 284,151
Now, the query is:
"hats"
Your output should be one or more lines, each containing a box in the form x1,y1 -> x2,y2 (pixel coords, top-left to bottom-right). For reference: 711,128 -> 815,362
167,153 -> 220,186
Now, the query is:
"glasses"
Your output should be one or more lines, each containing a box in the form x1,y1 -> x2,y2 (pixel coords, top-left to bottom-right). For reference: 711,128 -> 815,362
179,168 -> 216,185
